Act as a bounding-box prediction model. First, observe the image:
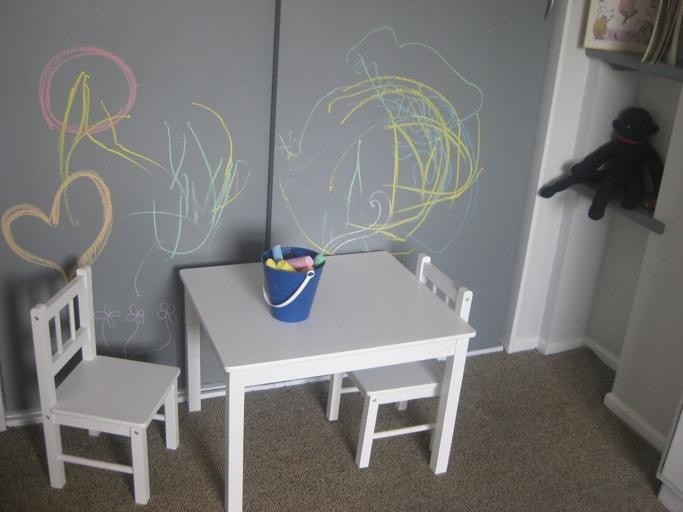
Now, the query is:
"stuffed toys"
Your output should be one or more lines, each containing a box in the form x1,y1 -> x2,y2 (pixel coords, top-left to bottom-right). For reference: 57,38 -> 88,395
539,108 -> 664,220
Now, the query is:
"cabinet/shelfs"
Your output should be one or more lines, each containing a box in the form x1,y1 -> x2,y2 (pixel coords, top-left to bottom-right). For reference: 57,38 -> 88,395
537,0 -> 683,453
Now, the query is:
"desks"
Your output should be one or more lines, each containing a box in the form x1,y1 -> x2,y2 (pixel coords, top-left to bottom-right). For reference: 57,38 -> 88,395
176,246 -> 474,512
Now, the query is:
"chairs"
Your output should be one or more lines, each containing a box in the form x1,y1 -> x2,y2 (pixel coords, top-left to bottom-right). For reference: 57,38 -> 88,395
320,250 -> 472,474
25,258 -> 184,509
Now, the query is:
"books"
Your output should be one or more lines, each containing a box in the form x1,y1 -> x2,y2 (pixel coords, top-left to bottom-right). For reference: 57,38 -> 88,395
582,0 -> 683,65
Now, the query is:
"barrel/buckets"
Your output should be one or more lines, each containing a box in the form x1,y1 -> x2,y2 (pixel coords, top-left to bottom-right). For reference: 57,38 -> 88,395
261,247 -> 324,322
